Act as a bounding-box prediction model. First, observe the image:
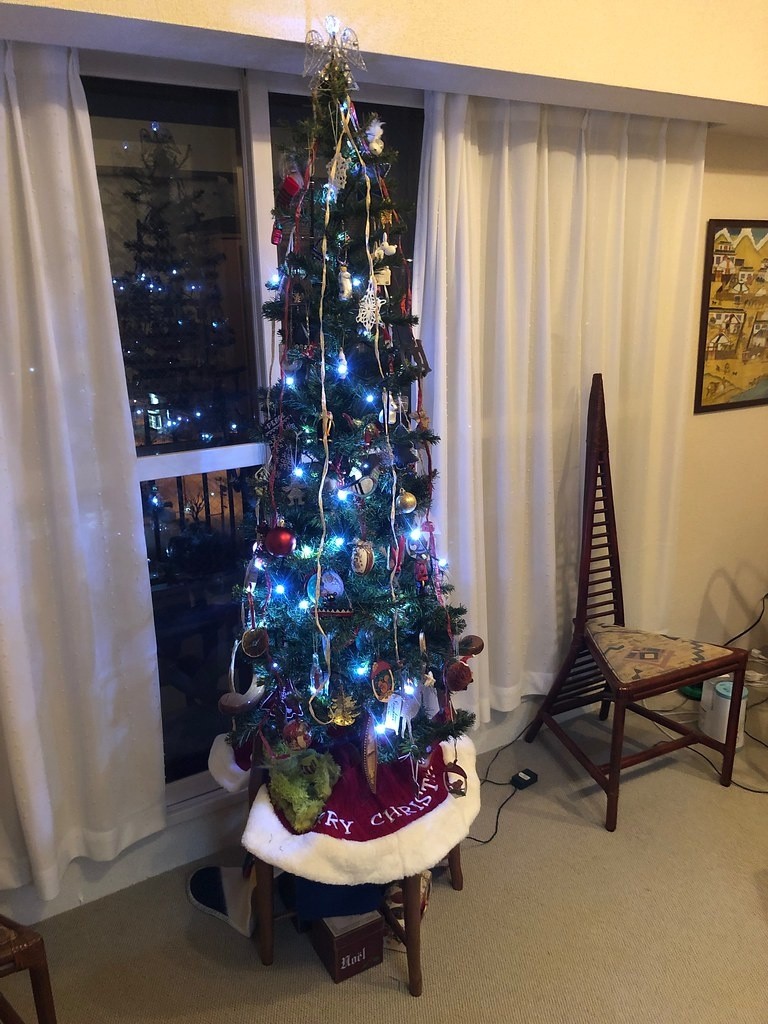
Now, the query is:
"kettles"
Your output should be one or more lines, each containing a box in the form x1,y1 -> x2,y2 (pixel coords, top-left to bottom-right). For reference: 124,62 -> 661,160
699,672 -> 750,751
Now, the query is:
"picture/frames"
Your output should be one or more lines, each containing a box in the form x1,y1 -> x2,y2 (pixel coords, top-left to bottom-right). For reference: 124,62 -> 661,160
694,218 -> 768,412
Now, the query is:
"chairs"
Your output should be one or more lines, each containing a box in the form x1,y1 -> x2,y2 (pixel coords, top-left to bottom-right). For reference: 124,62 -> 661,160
525,370 -> 748,831
248,746 -> 464,998
0,909 -> 55,1022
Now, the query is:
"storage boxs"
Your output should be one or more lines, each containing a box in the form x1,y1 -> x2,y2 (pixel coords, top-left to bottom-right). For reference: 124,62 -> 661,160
311,912 -> 388,983
